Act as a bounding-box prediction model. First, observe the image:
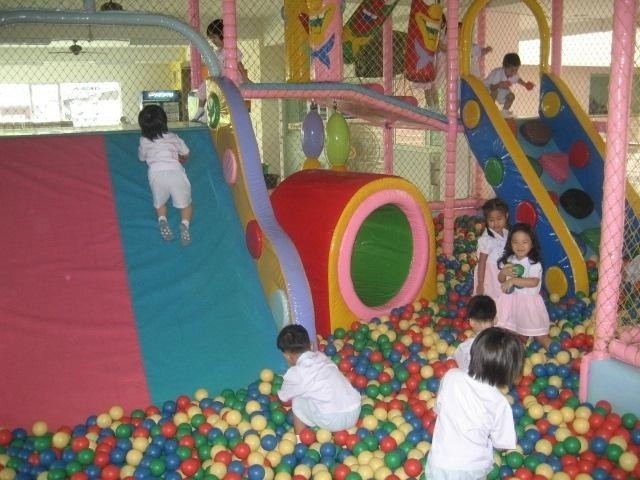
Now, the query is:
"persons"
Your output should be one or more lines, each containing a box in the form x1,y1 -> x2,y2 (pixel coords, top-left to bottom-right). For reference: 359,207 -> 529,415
473,199 -> 511,297
497,223 -> 550,342
138,105 -> 192,247
455,296 -> 497,369
481,54 -> 535,122
422,326 -> 522,480
272,324 -> 362,445
188,20 -> 246,122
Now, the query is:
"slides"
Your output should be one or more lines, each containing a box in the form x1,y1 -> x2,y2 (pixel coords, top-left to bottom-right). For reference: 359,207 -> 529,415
461,72 -> 640,298
0,77 -> 319,433
